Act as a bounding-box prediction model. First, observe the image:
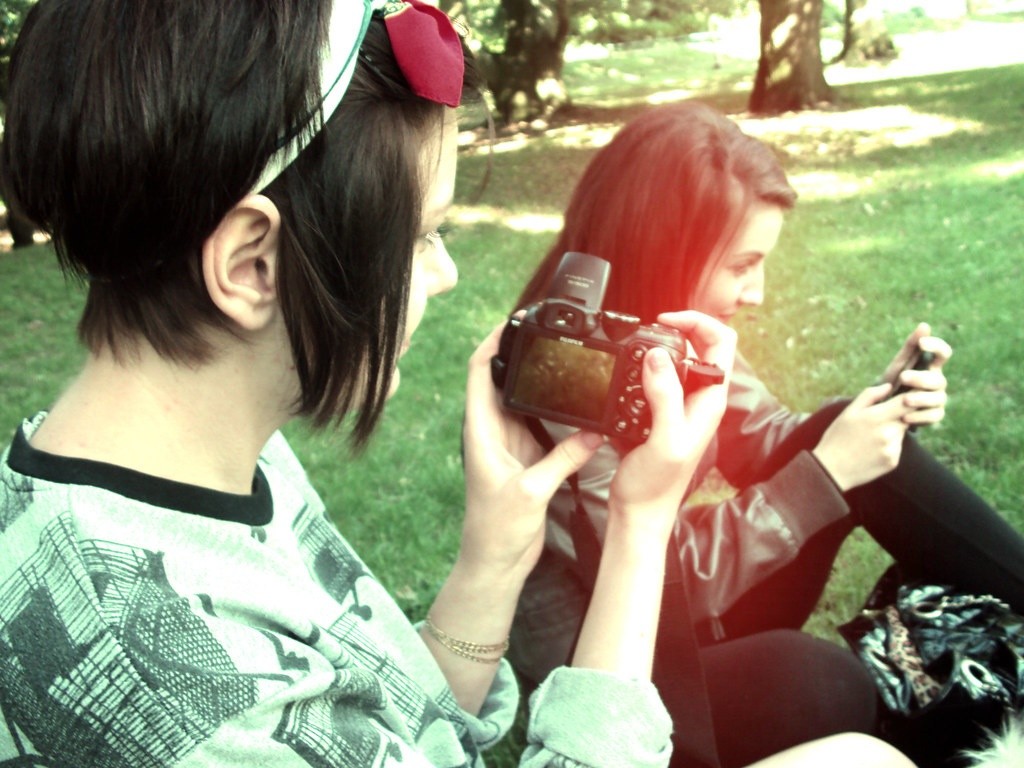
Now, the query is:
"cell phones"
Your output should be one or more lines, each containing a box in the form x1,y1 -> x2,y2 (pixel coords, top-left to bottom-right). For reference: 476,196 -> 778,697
890,342 -> 935,401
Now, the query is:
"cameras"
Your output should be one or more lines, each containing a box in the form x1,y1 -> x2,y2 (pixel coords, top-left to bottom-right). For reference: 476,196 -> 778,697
490,251 -> 689,445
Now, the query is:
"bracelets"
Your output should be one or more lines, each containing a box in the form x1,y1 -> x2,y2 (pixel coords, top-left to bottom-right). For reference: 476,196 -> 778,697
425,614 -> 509,665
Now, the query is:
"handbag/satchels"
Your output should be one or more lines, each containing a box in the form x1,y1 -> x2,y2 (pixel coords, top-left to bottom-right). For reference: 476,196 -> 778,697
836,564 -> 1024,714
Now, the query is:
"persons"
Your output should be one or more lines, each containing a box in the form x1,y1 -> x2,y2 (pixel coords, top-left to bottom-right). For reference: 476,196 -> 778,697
0,0 -> 916,768
507,100 -> 1024,768
